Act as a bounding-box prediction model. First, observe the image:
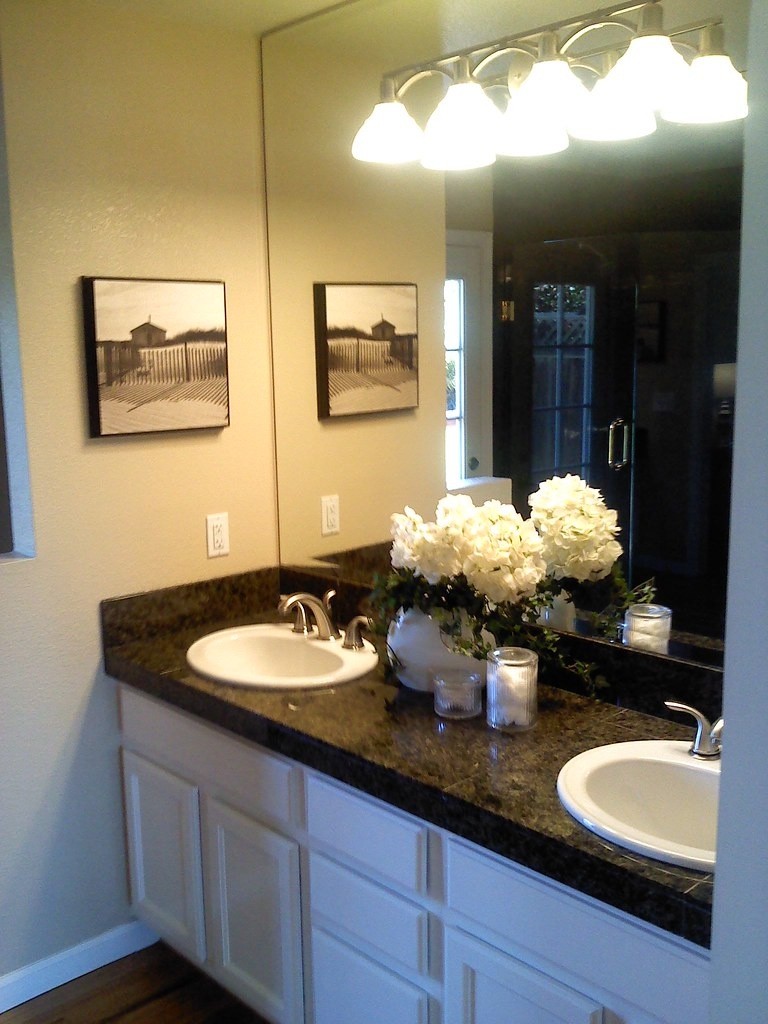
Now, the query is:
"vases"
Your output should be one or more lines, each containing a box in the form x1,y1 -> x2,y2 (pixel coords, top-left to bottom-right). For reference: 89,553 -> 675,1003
387,604 -> 496,692
525,588 -> 577,632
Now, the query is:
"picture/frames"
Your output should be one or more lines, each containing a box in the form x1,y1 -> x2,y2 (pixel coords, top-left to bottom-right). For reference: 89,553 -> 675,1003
313,282 -> 419,418
82,275 -> 231,438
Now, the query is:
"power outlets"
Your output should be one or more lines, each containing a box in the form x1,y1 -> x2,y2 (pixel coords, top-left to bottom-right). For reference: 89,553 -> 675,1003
320,493 -> 339,536
206,512 -> 230,558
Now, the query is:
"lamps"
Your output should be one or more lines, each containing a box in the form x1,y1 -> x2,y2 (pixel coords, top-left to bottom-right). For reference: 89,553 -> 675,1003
351,0 -> 748,171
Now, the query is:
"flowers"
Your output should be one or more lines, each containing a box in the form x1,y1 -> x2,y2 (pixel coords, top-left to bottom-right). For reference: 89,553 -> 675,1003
525,474 -> 657,646
367,490 -> 598,711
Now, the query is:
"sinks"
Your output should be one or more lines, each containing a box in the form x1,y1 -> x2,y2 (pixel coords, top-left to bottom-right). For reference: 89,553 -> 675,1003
184,622 -> 380,689
558,739 -> 722,875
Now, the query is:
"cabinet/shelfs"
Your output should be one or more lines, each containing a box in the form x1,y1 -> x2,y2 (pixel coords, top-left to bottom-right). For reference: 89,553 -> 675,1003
116,686 -> 711,1024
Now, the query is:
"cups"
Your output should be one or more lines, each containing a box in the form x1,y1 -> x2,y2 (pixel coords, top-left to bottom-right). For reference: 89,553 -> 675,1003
431,667 -> 483,719
621,603 -> 673,656
485,646 -> 540,733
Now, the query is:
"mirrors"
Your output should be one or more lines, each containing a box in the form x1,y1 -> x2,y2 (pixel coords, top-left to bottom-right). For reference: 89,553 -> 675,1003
260,0 -> 748,673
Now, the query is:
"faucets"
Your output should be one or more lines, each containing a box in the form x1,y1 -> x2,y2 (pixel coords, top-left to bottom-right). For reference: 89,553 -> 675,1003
277,589 -> 341,641
707,716 -> 724,743
